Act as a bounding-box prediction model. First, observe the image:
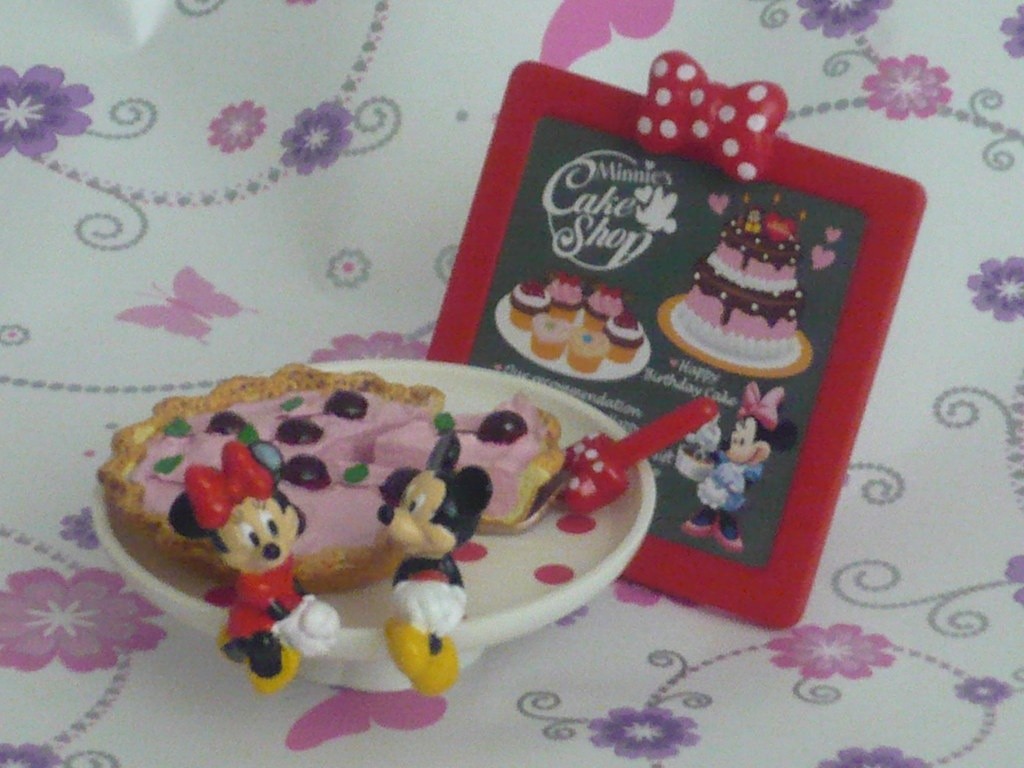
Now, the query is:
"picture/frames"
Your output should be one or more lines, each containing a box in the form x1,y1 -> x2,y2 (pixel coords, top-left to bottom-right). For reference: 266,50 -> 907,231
427,50 -> 928,628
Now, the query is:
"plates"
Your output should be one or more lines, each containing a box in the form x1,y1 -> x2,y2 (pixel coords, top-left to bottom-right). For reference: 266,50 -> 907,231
86,360 -> 657,693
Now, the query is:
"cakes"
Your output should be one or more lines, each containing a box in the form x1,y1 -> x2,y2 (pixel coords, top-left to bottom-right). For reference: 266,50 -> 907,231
98,364 -> 567,608
